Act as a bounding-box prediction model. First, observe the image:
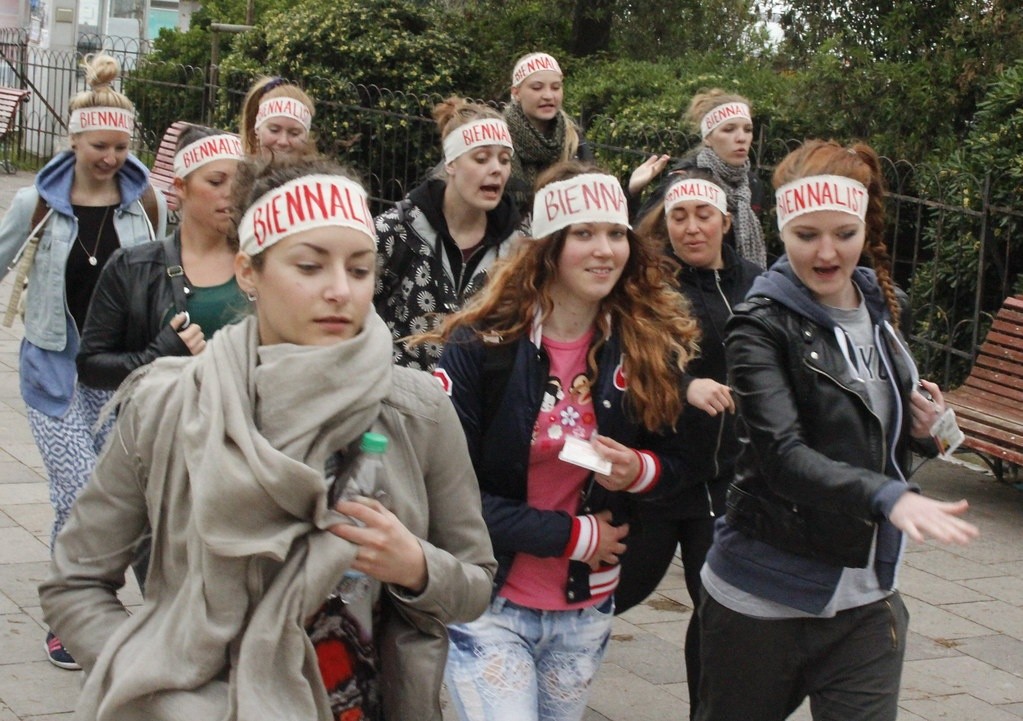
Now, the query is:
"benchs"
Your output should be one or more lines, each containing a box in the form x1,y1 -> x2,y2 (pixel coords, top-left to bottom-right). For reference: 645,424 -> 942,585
900,295 -> 1023,481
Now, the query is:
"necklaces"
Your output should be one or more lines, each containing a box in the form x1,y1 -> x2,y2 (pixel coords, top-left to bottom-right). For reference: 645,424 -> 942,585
77,193 -> 111,268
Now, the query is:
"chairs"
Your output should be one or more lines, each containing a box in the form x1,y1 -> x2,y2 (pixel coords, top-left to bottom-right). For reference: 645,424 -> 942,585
149,121 -> 245,209
0,86 -> 30,174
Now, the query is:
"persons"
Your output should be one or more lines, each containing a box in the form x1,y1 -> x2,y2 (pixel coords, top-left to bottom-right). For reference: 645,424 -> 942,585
238,78 -> 316,163
941,438 -> 950,451
0,49 -> 167,670
498,50 -> 601,247
636,86 -> 770,271
684,138 -> 978,721
368,95 -> 538,383
604,162 -> 766,615
430,161 -> 701,721
76,118 -> 259,395
37,156 -> 496,721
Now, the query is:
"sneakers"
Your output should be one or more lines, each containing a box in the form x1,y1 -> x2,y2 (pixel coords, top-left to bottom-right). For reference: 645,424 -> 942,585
45,630 -> 81,669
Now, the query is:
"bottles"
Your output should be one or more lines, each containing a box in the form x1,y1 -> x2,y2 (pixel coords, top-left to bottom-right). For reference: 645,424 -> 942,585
327,431 -> 388,604
